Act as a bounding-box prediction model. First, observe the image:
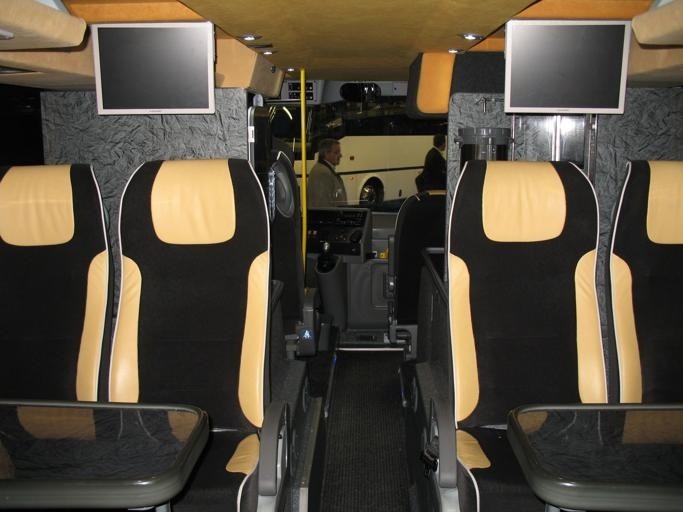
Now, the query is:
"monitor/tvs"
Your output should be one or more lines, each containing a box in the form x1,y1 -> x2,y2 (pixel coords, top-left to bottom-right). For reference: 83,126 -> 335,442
502,17 -> 632,115
89,20 -> 217,115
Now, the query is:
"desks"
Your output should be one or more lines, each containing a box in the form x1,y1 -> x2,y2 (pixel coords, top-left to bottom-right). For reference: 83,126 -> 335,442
505,404 -> 683,512
1,399 -> 210,512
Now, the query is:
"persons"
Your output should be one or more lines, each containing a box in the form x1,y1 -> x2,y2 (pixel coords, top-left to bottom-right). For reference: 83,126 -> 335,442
415,132 -> 447,193
306,138 -> 347,207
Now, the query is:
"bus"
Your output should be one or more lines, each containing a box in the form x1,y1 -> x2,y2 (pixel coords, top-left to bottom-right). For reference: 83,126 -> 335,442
267,99 -> 448,211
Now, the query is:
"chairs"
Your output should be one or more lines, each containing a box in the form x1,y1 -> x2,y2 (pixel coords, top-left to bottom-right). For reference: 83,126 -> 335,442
246,105 -> 340,395
437,160 -> 634,511
384,190 -> 447,463
98,160 -> 292,512
0,164 -> 115,484
608,160 -> 683,404
